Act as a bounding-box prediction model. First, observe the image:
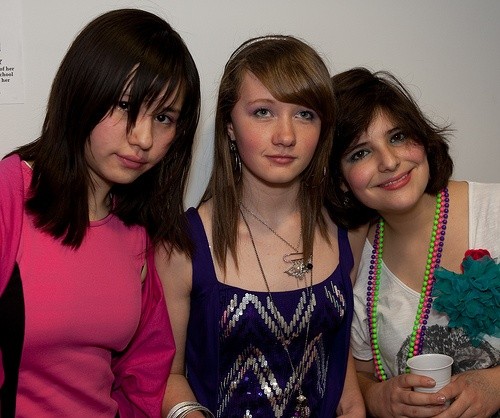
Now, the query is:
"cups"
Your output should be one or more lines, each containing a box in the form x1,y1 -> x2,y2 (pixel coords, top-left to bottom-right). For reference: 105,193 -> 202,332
406,354 -> 454,408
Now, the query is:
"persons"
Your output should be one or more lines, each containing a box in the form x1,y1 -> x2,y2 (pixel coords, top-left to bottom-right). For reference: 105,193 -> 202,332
0,8 -> 202,418
330,68 -> 500,418
153,36 -> 371,418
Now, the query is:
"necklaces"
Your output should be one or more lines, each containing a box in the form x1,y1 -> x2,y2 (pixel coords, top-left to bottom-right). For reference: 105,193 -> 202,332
366,188 -> 450,391
240,199 -> 315,404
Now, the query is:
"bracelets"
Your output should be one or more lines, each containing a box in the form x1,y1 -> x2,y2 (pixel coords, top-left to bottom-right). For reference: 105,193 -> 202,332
166,402 -> 214,418
